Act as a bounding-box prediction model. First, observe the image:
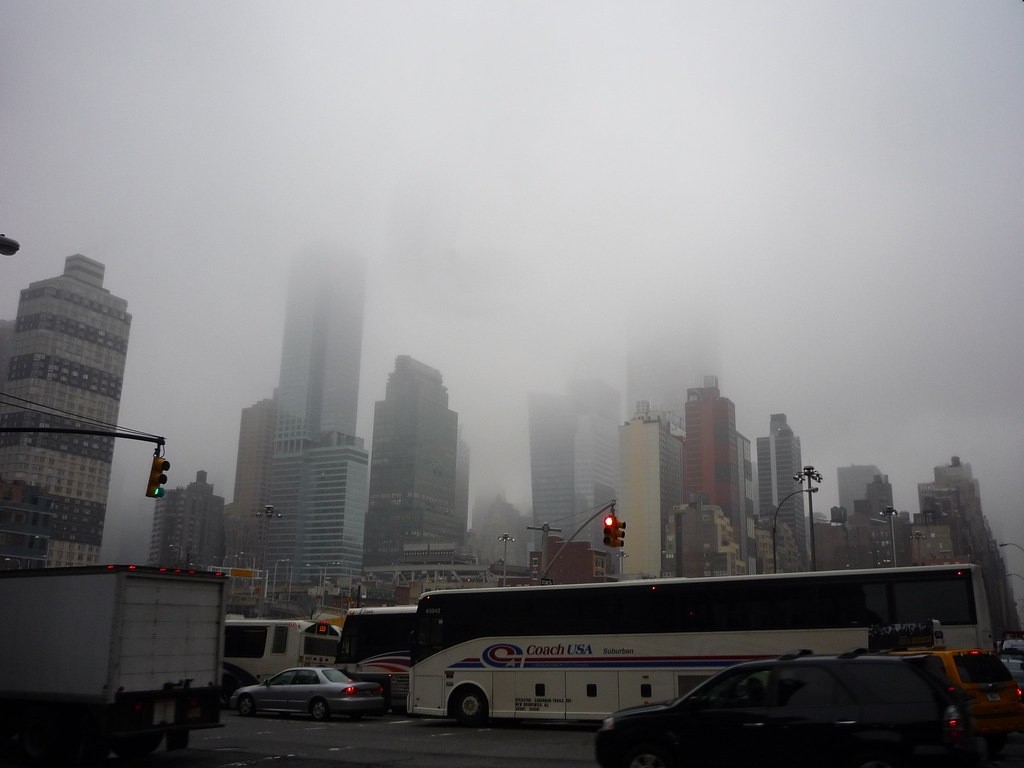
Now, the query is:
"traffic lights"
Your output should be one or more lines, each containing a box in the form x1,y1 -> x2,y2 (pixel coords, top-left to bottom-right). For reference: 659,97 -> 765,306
603,516 -> 615,546
145,456 -> 170,498
612,515 -> 626,548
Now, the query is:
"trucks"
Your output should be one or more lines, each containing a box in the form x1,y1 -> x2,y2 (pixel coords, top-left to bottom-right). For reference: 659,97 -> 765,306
0,563 -> 231,768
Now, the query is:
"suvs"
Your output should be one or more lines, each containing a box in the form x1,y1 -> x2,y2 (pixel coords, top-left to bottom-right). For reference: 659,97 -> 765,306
870,644 -> 1024,757
592,644 -> 991,768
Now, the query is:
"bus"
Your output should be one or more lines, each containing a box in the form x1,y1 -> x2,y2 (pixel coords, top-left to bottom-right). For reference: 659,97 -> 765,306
337,603 -> 416,717
410,562 -> 995,730
216,611 -> 341,711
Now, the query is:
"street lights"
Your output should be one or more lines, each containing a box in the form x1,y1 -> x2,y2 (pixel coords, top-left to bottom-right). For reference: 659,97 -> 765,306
322,563 -> 337,606
498,532 -> 515,587
5,556 -> 22,570
772,486 -> 819,574
793,465 -> 824,572
306,563 -> 322,601
34,535 -> 50,568
241,551 -> 257,600
256,503 -> 284,620
908,531 -> 928,564
881,506 -> 899,568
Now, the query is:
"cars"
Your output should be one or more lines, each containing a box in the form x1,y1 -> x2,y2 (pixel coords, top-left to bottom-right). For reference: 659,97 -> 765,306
230,667 -> 386,723
995,627 -> 1024,684
